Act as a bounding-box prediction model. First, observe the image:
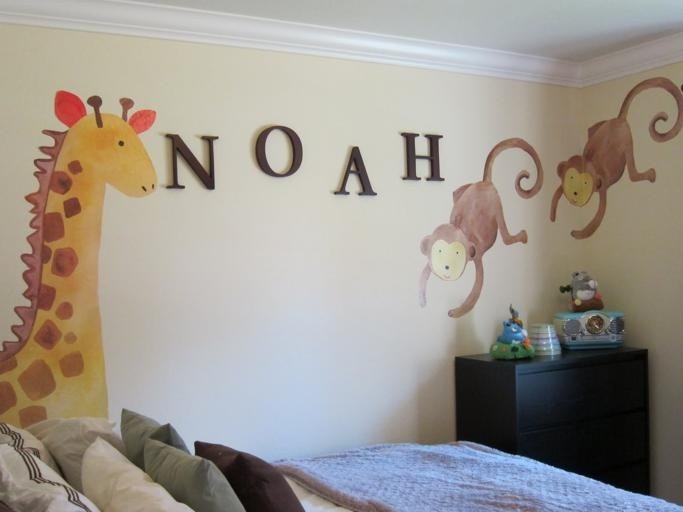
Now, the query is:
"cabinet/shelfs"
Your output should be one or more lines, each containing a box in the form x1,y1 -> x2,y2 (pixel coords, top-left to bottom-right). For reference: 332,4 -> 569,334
455,344 -> 649,496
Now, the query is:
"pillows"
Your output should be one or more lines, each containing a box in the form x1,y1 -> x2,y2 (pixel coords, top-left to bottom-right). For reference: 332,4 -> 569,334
142,437 -> 245,508
73,434 -> 190,508
191,437 -> 303,508
118,409 -> 201,471
1,420 -> 97,508
22,414 -> 134,496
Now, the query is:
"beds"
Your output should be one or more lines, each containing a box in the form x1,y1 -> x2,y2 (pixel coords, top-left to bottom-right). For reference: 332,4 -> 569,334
0,442 -> 683,511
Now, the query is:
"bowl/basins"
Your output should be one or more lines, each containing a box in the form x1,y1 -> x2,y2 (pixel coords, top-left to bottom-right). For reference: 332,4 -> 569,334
527,324 -> 563,356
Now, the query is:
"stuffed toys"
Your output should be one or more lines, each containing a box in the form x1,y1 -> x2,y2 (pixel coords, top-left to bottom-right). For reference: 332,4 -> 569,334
558,270 -> 603,311
